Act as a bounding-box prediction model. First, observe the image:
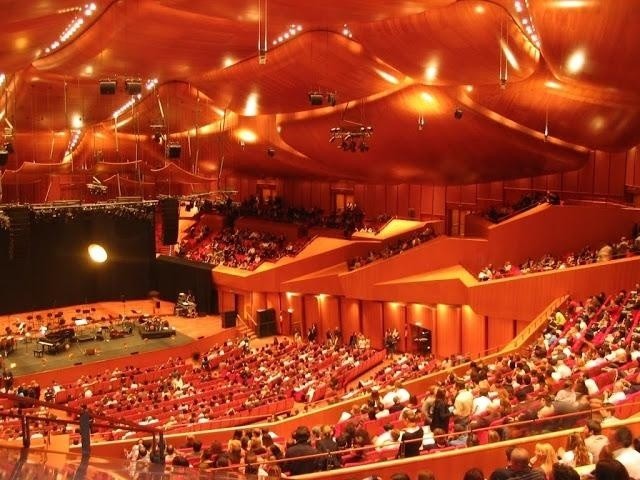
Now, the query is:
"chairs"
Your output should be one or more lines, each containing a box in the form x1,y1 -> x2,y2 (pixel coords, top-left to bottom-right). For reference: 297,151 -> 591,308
0,289 -> 640,480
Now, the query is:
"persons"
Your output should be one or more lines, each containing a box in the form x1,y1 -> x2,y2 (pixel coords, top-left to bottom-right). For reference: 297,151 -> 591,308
0,191 -> 640,480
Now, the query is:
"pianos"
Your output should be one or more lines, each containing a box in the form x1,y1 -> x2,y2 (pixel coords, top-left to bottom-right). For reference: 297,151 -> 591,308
36,328 -> 74,355
